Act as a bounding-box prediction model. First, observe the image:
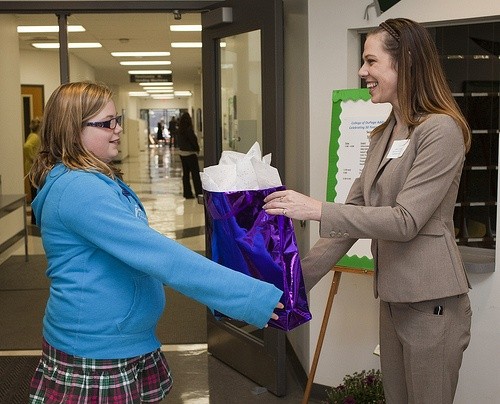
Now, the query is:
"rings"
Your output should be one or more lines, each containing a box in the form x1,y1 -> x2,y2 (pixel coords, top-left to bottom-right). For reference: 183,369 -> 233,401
279,196 -> 284,201
282,209 -> 287,215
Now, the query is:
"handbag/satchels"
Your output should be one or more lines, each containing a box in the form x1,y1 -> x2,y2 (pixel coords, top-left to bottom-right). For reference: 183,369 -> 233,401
201,186 -> 313,331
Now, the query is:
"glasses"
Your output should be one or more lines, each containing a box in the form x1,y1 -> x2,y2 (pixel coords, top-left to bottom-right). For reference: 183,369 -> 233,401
83,116 -> 122,129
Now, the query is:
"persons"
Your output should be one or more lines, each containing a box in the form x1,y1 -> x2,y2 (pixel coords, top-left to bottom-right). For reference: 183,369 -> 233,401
156,112 -> 203,200
262,18 -> 472,404
24,116 -> 43,224
25,81 -> 283,404
